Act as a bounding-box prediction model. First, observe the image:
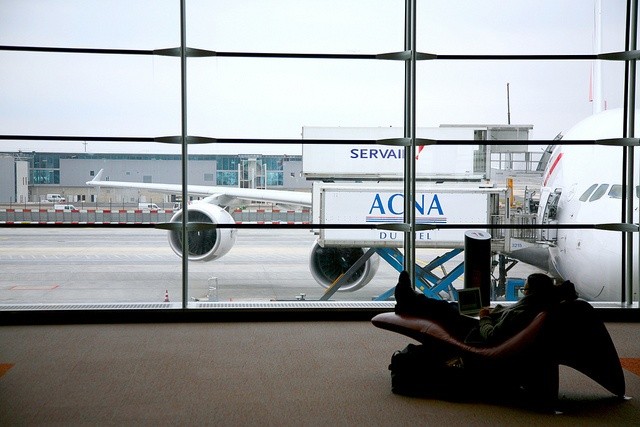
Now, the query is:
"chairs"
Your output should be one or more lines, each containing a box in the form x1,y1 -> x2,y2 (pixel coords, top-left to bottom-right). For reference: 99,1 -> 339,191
372,298 -> 627,405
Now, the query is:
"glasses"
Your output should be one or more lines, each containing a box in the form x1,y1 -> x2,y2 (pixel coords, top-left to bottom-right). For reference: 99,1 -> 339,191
520,288 -> 529,294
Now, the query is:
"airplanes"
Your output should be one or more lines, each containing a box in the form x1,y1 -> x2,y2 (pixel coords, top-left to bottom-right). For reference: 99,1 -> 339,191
86,0 -> 640,301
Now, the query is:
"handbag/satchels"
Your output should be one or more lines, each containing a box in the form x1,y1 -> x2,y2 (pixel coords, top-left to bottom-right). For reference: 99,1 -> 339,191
389,344 -> 426,396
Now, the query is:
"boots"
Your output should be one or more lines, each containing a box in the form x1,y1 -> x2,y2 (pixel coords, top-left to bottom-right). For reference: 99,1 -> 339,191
395,271 -> 425,317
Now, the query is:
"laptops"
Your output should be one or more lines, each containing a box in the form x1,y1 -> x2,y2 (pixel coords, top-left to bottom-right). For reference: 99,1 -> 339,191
458,288 -> 482,321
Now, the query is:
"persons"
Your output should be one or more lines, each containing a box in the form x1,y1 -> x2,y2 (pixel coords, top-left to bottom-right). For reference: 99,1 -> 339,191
394,270 -> 553,348
